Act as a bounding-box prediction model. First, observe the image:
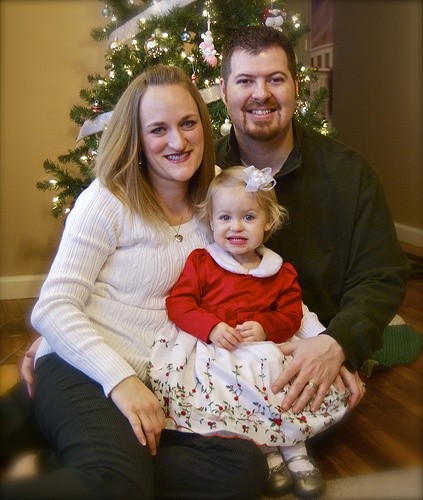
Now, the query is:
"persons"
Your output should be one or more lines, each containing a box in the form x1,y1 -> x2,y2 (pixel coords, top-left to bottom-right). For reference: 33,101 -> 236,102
1,63 -> 365,500
20,28 -> 423,414
150,166 -> 350,500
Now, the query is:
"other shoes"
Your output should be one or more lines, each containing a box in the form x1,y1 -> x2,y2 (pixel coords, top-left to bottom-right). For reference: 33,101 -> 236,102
264,451 -> 294,498
287,454 -> 326,500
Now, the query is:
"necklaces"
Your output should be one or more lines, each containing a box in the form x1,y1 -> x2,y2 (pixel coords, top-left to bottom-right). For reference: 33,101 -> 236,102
170,216 -> 183,242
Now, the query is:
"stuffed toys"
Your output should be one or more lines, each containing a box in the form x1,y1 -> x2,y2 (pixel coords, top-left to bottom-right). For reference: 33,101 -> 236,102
199,31 -> 217,67
264,9 -> 285,32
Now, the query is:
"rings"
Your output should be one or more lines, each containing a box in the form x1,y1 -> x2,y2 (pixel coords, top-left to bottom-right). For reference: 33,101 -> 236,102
361,382 -> 365,386
308,382 -> 318,389
